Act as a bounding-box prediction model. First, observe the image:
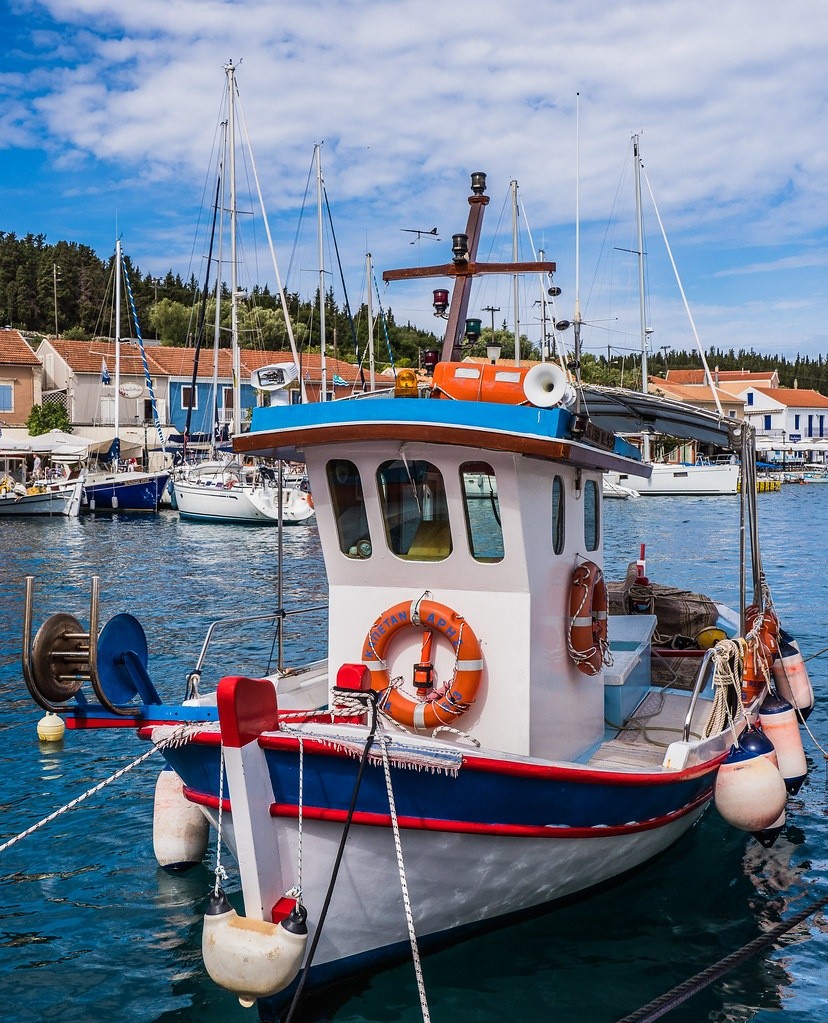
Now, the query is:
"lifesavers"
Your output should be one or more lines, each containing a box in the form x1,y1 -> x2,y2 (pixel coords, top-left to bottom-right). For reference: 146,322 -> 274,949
569,562 -> 608,675
363,600 -> 483,728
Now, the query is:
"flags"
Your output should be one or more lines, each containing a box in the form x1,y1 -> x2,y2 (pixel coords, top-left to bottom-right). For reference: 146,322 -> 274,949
101,357 -> 112,387
334,373 -> 349,387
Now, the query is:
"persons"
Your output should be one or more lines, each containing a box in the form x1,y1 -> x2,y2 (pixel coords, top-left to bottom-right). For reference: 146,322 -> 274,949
32,453 -> 42,482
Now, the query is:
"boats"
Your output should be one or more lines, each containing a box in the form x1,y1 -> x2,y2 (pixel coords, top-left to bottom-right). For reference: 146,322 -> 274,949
58,166 -> 814,1010
7,51 -> 828,529
